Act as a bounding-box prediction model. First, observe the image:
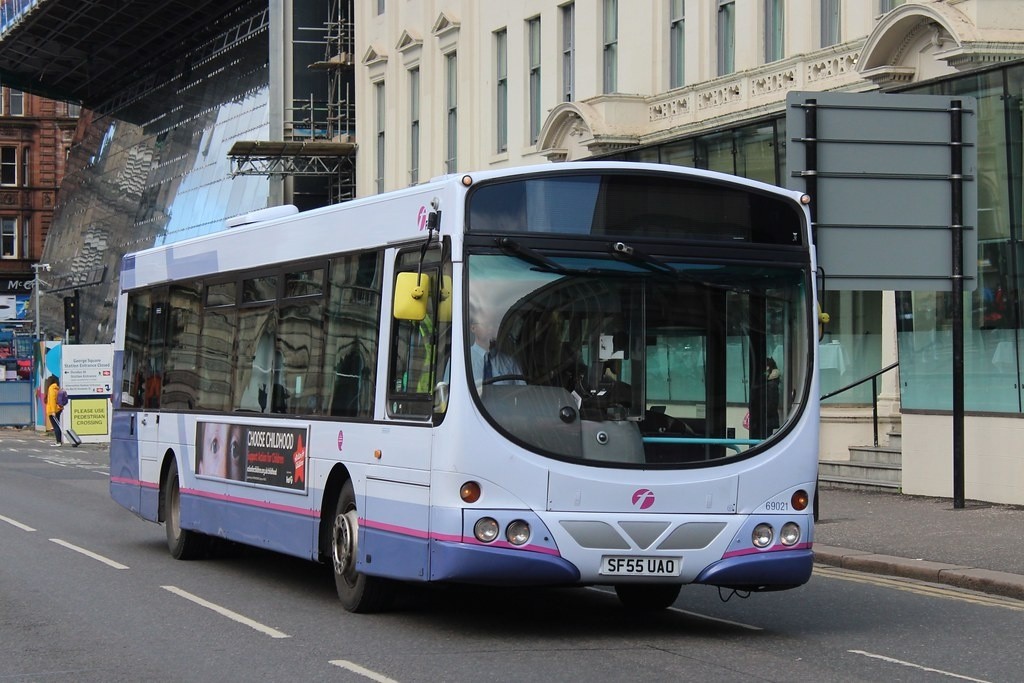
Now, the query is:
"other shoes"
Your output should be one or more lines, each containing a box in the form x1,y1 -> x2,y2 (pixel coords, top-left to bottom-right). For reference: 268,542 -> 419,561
52,443 -> 62,447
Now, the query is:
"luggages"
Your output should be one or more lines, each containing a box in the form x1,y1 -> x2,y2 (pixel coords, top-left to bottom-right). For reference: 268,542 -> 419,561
52,416 -> 81,447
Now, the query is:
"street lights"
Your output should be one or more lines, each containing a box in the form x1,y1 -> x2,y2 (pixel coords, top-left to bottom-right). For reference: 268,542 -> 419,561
31,263 -> 51,340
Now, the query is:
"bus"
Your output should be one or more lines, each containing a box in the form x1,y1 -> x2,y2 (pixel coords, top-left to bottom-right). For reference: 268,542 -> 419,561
107,161 -> 831,615
12,330 -> 46,379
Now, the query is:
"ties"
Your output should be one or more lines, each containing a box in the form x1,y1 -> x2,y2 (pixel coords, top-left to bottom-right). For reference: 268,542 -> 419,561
482,354 -> 492,385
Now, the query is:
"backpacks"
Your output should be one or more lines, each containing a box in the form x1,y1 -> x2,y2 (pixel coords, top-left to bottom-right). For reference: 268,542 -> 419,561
54,384 -> 68,408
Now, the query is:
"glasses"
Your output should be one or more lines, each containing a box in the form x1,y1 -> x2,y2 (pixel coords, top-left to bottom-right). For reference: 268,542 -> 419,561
472,320 -> 502,329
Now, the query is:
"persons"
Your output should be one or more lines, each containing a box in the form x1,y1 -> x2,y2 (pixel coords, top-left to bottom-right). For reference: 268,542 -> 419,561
765,356 -> 780,440
45,375 -> 64,446
471,310 -> 527,390
